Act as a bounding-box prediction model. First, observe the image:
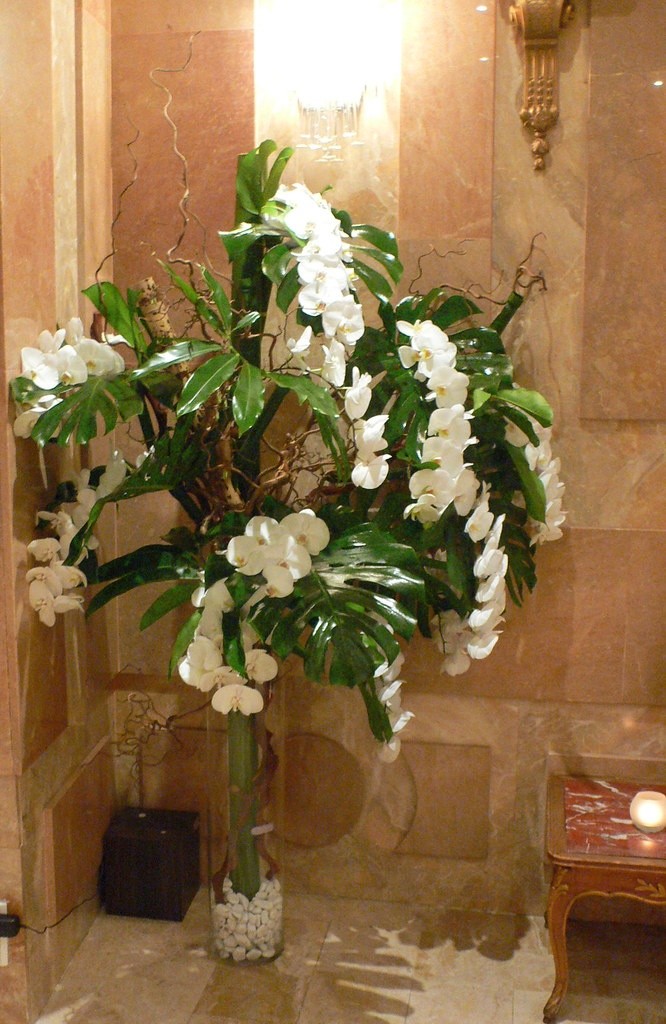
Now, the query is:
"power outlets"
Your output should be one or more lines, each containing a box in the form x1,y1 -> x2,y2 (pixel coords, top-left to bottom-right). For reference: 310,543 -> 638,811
0,901 -> 9,967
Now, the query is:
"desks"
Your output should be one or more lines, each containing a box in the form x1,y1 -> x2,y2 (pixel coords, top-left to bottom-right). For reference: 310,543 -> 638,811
543,772 -> 666,1024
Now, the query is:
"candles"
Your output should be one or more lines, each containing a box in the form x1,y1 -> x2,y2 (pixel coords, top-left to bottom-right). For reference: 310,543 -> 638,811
630,791 -> 666,833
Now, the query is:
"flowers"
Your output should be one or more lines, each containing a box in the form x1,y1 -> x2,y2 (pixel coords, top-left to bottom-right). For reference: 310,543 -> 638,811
8,139 -> 569,764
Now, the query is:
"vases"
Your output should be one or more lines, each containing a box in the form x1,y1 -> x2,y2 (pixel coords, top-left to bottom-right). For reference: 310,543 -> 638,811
207,677 -> 286,968
